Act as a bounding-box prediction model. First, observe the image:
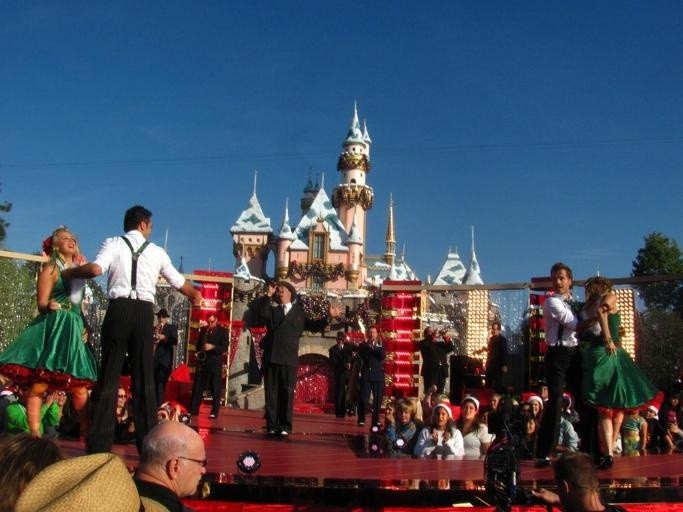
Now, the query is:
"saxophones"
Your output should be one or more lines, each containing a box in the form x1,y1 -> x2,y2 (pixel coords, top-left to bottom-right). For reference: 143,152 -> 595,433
193,326 -> 211,364
152,323 -> 162,343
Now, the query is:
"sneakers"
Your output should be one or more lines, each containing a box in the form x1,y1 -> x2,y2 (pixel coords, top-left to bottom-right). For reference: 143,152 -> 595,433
358,422 -> 365,426
373,423 -> 382,427
267,430 -> 289,438
209,414 -> 216,418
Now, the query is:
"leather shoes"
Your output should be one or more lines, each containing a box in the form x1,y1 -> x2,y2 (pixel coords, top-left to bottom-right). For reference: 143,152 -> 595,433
595,455 -> 614,468
535,457 -> 555,467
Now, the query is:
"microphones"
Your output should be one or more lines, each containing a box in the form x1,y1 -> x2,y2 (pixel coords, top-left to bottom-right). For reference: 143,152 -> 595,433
261,272 -> 279,291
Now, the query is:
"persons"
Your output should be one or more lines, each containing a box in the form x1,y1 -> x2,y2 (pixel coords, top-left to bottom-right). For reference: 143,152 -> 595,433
61,204 -> 203,456
531,452 -> 627,511
575,276 -> 660,467
0,224 -> 86,446
131,419 -> 207,512
246,280 -> 344,440
534,262 -> 596,465
2,304 -> 682,492
0,435 -> 60,512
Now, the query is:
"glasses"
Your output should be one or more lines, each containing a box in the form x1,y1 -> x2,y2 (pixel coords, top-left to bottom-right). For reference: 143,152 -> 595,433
118,395 -> 127,398
178,457 -> 209,466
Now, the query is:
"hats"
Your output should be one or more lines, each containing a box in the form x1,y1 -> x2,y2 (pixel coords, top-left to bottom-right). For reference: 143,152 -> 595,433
15,451 -> 170,512
647,399 -> 660,414
430,402 -> 453,425
154,308 -> 170,317
520,393 -> 544,410
460,393 -> 480,411
272,281 -> 297,304
